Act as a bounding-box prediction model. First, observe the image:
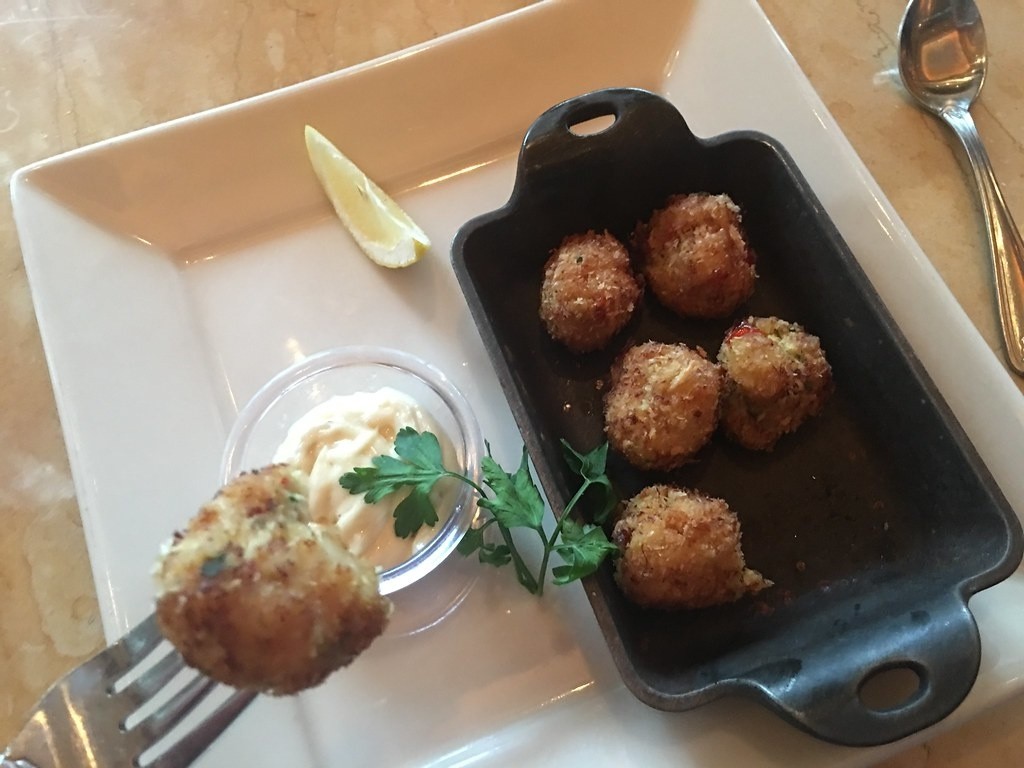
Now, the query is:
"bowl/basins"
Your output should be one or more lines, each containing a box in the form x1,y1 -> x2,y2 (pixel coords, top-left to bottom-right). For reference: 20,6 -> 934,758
221,346 -> 481,612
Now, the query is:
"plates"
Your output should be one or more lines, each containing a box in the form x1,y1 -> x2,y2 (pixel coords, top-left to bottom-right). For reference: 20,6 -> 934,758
8,0 -> 1024,768
450,78 -> 1022,746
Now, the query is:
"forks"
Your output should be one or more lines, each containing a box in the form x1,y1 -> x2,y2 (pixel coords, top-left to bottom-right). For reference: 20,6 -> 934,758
0,550 -> 335,767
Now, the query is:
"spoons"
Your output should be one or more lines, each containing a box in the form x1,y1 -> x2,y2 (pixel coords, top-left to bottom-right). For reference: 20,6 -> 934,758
894,1 -> 1024,374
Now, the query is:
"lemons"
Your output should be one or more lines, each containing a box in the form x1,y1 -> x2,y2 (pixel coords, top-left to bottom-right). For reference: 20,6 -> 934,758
304,123 -> 429,269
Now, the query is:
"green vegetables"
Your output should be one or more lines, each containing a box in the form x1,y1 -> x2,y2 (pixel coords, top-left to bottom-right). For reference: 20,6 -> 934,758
339,427 -> 617,596
